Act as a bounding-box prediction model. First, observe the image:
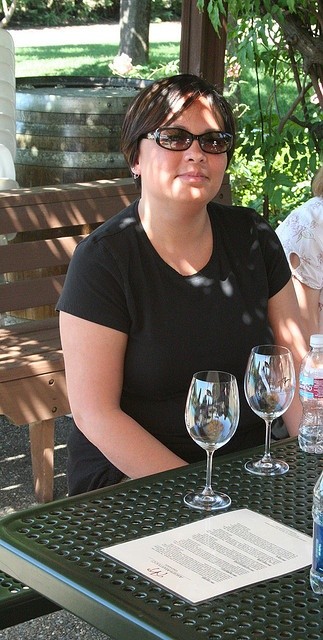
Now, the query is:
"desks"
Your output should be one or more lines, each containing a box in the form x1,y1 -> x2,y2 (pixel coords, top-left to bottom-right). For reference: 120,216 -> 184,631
1,433 -> 323,640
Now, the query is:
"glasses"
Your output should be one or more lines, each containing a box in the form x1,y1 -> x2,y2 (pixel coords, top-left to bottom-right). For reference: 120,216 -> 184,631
139,127 -> 234,154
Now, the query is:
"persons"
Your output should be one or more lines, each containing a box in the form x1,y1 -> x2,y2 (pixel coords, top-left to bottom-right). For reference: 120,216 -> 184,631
56,74 -> 314,498
272,168 -> 322,440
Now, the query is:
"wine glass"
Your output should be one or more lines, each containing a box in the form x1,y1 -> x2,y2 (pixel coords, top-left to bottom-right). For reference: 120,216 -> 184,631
243,344 -> 296,477
184,370 -> 240,511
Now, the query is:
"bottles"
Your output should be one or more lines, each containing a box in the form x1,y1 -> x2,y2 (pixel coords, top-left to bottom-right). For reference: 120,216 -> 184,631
297,333 -> 322,454
308,470 -> 323,595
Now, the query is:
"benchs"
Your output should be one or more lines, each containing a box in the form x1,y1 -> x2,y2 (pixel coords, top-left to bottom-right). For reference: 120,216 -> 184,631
1,172 -> 233,503
1,569 -> 65,629
